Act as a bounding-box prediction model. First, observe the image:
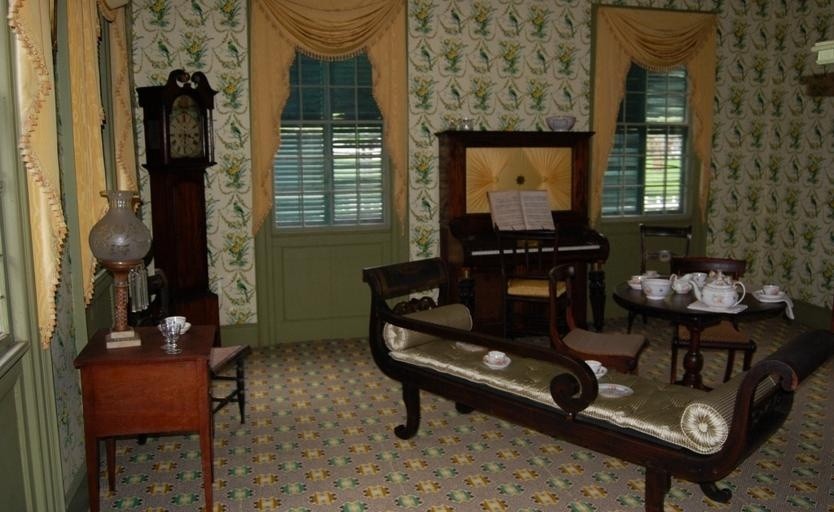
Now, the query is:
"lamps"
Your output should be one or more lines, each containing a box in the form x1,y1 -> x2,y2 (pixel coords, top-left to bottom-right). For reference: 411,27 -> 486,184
88,191 -> 151,351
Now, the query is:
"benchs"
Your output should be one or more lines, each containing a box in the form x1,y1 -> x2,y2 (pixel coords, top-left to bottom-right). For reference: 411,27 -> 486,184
361,257 -> 834,512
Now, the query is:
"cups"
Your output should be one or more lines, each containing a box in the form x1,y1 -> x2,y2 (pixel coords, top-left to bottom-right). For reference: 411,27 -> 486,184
460,111 -> 474,130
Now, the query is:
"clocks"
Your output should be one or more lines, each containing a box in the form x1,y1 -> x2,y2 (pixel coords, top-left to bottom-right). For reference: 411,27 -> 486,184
136,69 -> 221,351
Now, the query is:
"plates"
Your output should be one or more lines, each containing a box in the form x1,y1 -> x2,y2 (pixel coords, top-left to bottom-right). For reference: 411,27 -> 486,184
756,291 -> 785,300
591,366 -> 608,381
157,322 -> 192,335
482,354 -> 512,371
643,274 -> 660,279
598,383 -> 635,400
627,280 -> 641,290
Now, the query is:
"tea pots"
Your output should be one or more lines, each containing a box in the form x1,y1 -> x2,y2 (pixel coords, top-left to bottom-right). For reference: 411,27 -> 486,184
687,277 -> 746,308
668,273 -> 693,295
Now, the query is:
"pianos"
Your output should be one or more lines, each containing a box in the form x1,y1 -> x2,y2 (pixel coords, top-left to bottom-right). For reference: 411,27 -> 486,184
436,131 -> 610,338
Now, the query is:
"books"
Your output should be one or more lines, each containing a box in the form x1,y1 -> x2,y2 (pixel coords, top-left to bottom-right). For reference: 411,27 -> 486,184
485,188 -> 558,233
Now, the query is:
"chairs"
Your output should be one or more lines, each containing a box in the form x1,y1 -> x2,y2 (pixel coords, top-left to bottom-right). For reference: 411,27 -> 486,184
550,264 -> 646,376
671,258 -> 757,394
494,225 -> 578,341
125,271 -> 250,444
626,222 -> 694,336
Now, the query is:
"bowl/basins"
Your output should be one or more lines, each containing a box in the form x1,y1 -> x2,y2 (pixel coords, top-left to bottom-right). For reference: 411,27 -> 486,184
584,360 -> 603,374
546,115 -> 576,132
762,285 -> 780,295
646,271 -> 656,276
693,273 -> 708,282
641,279 -> 673,301
165,316 -> 187,329
488,351 -> 505,365
631,276 -> 648,284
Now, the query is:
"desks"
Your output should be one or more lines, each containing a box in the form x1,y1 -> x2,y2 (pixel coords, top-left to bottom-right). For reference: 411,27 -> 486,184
75,323 -> 218,512
613,277 -> 788,394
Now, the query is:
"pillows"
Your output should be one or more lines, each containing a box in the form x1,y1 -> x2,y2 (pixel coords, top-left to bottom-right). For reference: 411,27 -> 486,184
384,303 -> 473,352
680,364 -> 776,450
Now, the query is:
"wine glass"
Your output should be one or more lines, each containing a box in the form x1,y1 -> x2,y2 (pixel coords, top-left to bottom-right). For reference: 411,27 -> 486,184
160,320 -> 175,350
165,325 -> 181,355
